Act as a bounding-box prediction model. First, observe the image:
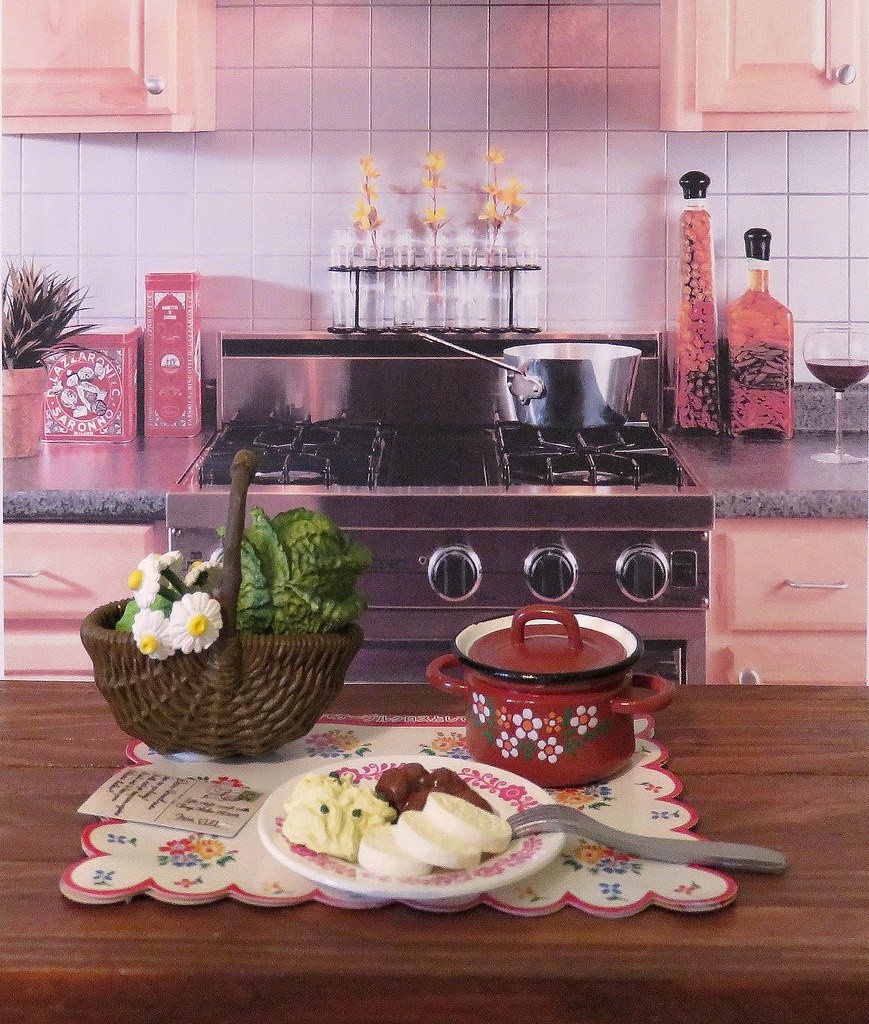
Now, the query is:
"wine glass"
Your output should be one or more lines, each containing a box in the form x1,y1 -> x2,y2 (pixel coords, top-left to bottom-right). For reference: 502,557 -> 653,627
801,324 -> 868,466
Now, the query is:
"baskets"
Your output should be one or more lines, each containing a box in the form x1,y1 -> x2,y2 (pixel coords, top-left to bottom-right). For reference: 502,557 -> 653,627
79,594 -> 365,759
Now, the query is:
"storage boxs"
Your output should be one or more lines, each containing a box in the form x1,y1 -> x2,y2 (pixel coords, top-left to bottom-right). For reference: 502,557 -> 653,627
40,325 -> 143,443
143,270 -> 202,438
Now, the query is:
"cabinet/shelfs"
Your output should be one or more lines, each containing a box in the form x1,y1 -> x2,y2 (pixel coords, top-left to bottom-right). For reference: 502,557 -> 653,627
1,0 -> 216,137
705,514 -> 869,688
4,517 -> 171,684
656,0 -> 868,133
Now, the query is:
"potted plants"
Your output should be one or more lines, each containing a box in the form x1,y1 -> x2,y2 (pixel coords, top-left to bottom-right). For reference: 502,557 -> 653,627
2,253 -> 116,460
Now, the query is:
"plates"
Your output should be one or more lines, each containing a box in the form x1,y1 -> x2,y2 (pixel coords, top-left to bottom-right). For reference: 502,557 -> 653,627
257,753 -> 567,899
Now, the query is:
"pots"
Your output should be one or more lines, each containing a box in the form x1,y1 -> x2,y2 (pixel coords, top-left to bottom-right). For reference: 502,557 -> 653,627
424,602 -> 677,790
411,331 -> 644,429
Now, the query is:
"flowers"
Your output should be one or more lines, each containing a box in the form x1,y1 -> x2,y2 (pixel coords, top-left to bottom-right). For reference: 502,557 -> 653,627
130,555 -> 161,609
155,548 -> 184,575
477,146 -> 530,249
422,149 -> 452,246
132,607 -> 175,661
168,590 -> 226,653
353,155 -> 386,254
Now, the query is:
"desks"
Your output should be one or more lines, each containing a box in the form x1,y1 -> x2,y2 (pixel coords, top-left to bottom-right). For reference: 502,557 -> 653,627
0,678 -> 869,1017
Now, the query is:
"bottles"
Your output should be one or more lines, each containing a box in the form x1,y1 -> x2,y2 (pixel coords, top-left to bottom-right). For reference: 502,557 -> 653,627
725,225 -> 794,442
329,229 -> 542,330
670,170 -> 721,437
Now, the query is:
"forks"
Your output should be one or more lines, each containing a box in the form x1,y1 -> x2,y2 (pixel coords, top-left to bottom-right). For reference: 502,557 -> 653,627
504,802 -> 787,874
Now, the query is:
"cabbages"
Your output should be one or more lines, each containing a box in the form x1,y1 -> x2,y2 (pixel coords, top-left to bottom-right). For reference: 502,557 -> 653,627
117,511 -> 372,637
